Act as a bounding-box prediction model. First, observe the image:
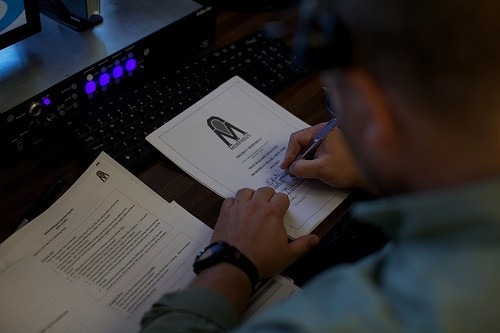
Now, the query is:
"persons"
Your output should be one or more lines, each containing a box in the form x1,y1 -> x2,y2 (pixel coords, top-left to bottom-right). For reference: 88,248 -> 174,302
139,0 -> 500,333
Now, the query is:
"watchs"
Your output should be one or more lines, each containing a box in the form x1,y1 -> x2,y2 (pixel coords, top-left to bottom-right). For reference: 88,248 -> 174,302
191,242 -> 260,295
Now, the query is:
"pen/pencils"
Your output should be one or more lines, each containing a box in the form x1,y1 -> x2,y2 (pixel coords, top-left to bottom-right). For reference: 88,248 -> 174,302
279,117 -> 339,180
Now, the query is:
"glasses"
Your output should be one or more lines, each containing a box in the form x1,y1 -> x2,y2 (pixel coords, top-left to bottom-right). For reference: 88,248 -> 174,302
326,97 -> 341,118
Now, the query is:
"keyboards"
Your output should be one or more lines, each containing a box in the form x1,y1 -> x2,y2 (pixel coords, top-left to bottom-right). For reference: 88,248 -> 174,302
63,29 -> 307,170
